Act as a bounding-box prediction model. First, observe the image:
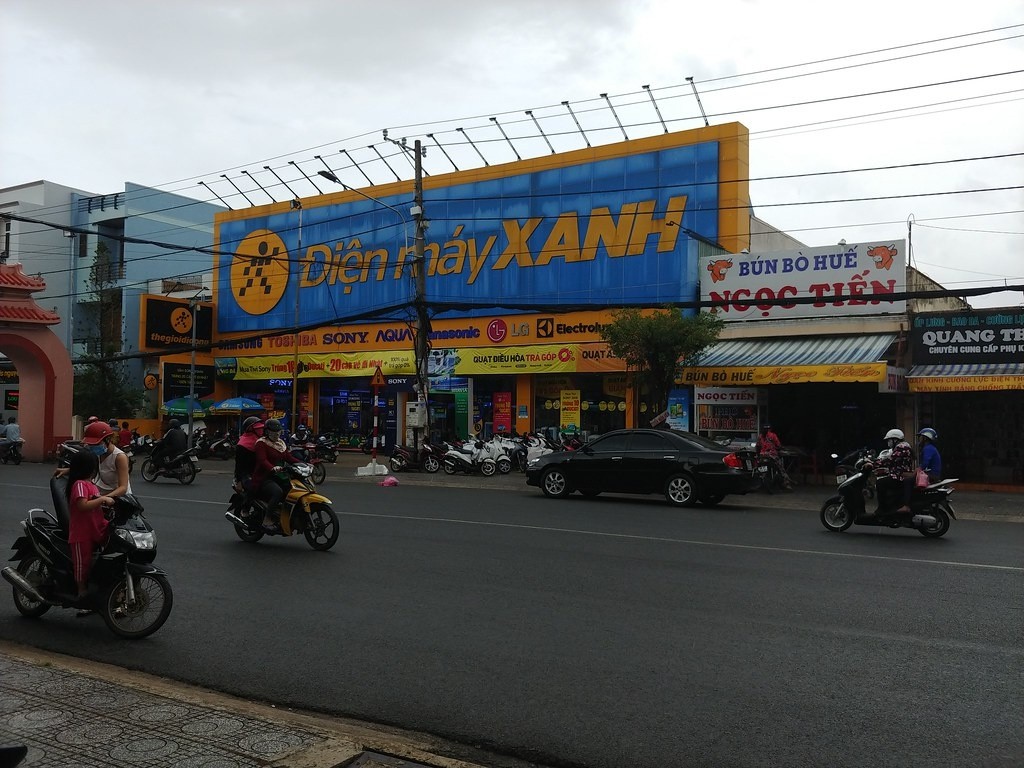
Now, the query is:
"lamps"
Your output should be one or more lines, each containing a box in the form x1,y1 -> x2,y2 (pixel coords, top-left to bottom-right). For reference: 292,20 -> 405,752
525,111 -> 555,153
288,161 -> 323,194
191,286 -> 210,300
740,248 -> 749,254
427,133 -> 459,171
339,149 -> 374,186
797,247 -> 808,255
642,85 -> 669,133
263,166 -> 300,198
665,221 -> 735,254
600,93 -> 629,140
560,100 -> 591,147
241,170 -> 277,203
166,281 -> 183,297
455,127 -> 490,166
489,117 -> 522,160
838,238 -> 846,252
219,174 -> 255,207
685,76 -> 709,126
393,141 -> 430,176
197,181 -> 233,211
314,155 -> 348,191
367,145 -> 401,181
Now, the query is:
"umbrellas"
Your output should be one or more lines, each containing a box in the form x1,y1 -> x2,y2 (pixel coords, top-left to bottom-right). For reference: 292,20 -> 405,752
160,398 -> 207,418
209,396 -> 265,438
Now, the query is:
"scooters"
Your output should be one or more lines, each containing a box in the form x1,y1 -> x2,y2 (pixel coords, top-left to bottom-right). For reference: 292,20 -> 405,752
284,429 -> 340,484
57,426 -> 158,475
819,448 -> 960,537
828,447 -> 870,488
361,430 -> 375,455
389,424 -> 587,476
192,426 -> 239,459
0,440 -> 25,466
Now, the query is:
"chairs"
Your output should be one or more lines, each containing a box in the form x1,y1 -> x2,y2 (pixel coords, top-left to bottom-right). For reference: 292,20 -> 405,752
799,452 -> 826,488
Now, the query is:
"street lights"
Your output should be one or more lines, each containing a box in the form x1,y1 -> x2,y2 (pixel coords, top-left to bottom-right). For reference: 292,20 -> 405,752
315,170 -> 430,460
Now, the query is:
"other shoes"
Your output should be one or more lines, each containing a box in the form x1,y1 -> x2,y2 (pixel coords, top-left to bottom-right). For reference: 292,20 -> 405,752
896,506 -> 912,513
261,522 -> 276,531
240,510 -> 252,521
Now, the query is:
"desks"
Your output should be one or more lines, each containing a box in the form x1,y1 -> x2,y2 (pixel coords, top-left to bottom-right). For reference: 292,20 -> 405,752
781,454 -> 800,473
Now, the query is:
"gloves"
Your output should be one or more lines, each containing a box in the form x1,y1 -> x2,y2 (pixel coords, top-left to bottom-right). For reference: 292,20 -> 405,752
271,466 -> 282,472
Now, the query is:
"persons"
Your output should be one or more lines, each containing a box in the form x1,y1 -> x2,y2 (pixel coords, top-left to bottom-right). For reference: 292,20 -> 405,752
234,416 -> 264,525
84,416 -> 98,431
118,422 -> 133,447
874,429 -> 912,517
897,428 -> 941,512
153,419 -> 187,476
0,416 -> 19,447
250,418 -> 301,531
55,421 -> 132,616
289,424 -> 310,444
755,423 -> 793,492
109,419 -> 121,432
66,451 -> 115,599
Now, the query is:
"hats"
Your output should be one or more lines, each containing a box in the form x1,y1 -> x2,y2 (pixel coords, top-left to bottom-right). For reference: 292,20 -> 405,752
249,420 -> 264,431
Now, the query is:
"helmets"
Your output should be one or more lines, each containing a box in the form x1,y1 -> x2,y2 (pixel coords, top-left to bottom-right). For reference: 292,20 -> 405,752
88,416 -> 98,421
81,422 -> 113,445
298,424 -> 306,430
9,416 -> 15,423
109,419 -> 118,425
264,418 -> 282,431
916,427 -> 938,442
170,419 -> 181,428
242,415 -> 264,431
882,428 -> 904,441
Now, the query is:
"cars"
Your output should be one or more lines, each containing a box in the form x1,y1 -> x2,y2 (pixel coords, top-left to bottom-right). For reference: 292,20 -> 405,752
524,428 -> 761,507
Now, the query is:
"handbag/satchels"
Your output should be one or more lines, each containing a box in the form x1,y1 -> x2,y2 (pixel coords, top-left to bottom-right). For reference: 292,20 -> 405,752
917,468 -> 929,487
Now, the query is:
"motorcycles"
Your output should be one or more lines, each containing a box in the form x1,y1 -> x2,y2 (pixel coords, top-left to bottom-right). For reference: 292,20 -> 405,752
141,442 -> 202,486
223,449 -> 339,552
1,474 -> 175,641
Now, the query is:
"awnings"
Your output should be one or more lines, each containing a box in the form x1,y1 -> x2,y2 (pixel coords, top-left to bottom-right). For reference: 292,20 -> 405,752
905,363 -> 1024,392
674,333 -> 897,385
